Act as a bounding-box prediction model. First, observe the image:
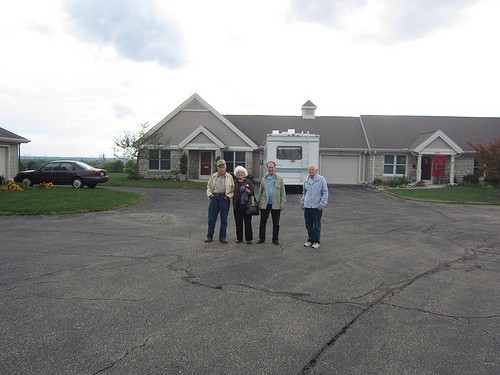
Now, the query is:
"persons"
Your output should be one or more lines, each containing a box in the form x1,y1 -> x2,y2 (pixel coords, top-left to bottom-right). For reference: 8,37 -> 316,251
231,165 -> 255,244
204,159 -> 234,243
300,163 -> 328,249
257,161 -> 286,245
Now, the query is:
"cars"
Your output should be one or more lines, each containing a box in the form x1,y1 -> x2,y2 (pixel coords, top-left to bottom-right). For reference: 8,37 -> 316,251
14,160 -> 109,189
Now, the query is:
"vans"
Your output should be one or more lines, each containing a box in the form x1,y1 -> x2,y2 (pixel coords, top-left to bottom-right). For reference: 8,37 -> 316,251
259,133 -> 320,186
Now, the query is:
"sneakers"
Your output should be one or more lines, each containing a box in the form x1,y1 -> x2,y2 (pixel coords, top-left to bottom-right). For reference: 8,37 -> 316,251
312,242 -> 320,249
304,242 -> 312,247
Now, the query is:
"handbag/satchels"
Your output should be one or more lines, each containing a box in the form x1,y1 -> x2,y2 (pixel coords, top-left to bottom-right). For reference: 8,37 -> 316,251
244,190 -> 259,216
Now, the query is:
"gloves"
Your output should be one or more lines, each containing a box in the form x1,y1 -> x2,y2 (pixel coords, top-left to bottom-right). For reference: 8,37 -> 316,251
239,186 -> 246,193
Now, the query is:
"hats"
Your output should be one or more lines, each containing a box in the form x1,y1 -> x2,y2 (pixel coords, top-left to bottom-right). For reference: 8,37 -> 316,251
217,159 -> 225,166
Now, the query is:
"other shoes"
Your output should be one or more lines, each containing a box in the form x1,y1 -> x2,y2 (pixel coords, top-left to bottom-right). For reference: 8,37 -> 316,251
204,237 -> 212,242
257,239 -> 264,244
247,240 -> 252,244
236,240 -> 243,243
219,237 -> 228,243
273,240 -> 279,245
